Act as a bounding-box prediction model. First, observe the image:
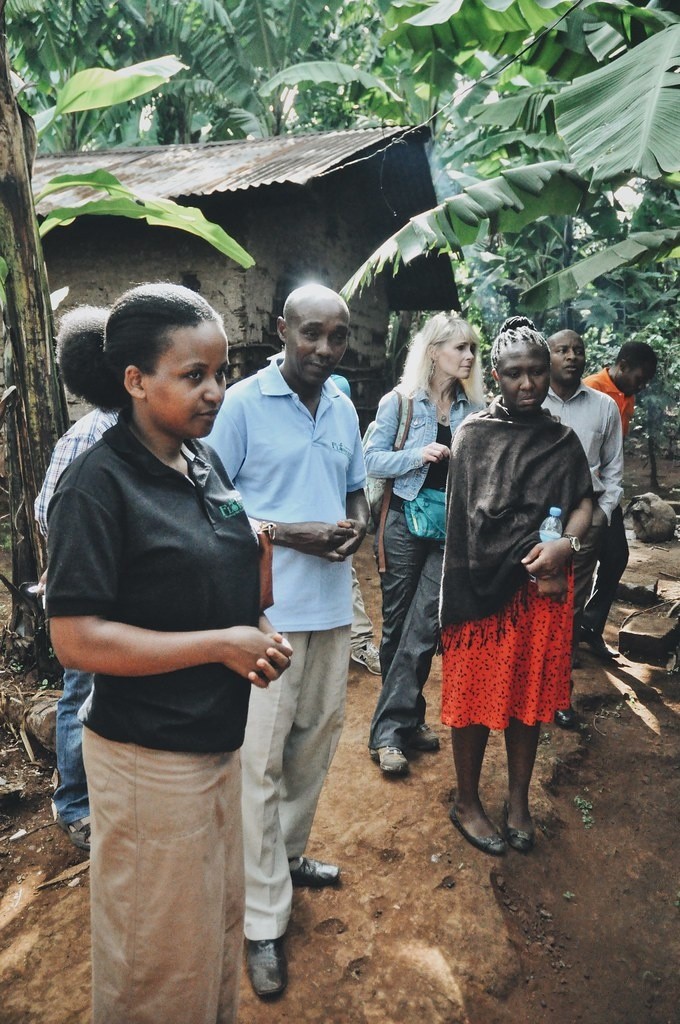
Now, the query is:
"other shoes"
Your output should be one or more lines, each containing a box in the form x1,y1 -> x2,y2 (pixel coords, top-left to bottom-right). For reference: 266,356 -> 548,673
580,626 -> 620,658
504,804 -> 534,851
450,807 -> 507,853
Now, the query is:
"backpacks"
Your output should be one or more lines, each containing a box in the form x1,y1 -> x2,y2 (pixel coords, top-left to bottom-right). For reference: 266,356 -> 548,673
362,390 -> 413,534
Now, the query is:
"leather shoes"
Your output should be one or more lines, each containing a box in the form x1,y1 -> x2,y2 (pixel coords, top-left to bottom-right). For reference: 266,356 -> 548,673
555,707 -> 576,728
288,856 -> 340,886
248,940 -> 286,994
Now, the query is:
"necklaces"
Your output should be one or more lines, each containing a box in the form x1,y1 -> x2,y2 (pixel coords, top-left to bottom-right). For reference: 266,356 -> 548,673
430,394 -> 450,422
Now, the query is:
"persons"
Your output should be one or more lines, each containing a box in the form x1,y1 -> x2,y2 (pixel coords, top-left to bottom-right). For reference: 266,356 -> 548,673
35,407 -> 119,851
45,283 -> 294,1024
540,329 -> 658,727
434,317 -> 593,855
361,314 -> 489,770
197,283 -> 370,996
332,374 -> 380,677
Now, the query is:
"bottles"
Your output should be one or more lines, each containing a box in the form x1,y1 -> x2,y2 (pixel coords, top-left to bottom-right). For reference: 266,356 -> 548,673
539,506 -> 563,543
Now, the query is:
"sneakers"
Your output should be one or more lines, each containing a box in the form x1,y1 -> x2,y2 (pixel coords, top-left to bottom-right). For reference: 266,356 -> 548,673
371,746 -> 408,772
402,724 -> 439,750
351,643 -> 382,674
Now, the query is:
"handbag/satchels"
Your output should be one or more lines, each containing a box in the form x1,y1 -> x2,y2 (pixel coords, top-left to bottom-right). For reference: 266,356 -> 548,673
403,488 -> 446,540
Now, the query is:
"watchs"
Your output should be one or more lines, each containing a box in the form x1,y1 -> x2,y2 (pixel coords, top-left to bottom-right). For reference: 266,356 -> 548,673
562,533 -> 581,553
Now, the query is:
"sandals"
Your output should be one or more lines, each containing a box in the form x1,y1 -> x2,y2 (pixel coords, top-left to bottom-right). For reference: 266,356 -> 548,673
52,802 -> 91,851
